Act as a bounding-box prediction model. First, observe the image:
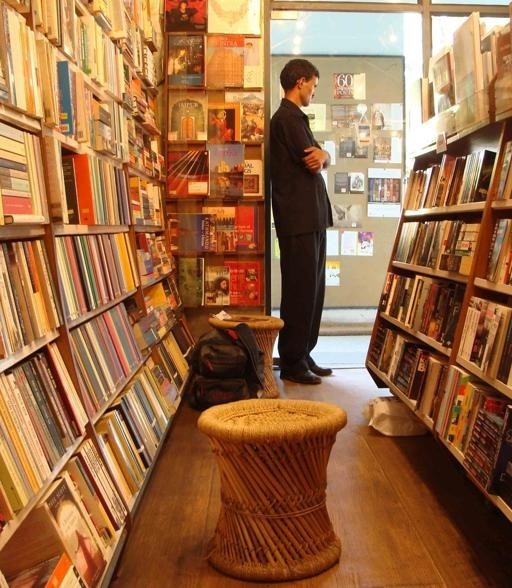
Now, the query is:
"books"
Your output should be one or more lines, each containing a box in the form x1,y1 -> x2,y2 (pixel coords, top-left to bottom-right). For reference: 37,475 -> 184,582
443,144 -> 512,494
0,1 -> 197,588
165,1 -> 264,306
396,220 -> 480,275
380,272 -> 465,347
403,149 -> 496,210
371,321 -> 446,417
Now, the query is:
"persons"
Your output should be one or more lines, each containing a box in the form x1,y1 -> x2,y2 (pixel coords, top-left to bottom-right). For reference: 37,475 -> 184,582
270,59 -> 332,384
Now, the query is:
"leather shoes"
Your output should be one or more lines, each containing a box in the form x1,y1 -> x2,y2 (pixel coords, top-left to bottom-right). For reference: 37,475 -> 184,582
280,367 -> 322,384
311,363 -> 332,377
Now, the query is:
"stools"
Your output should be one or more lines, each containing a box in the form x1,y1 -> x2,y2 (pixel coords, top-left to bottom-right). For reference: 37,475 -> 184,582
197,396 -> 348,579
208,312 -> 284,397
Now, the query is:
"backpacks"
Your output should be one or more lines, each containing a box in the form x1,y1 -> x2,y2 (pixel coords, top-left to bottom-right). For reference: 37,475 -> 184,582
187,323 -> 266,410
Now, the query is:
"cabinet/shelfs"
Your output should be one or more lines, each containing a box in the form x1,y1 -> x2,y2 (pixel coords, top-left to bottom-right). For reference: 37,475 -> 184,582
366,108 -> 512,519
0,0 -> 267,588
